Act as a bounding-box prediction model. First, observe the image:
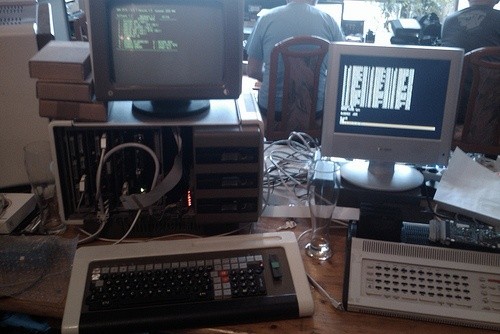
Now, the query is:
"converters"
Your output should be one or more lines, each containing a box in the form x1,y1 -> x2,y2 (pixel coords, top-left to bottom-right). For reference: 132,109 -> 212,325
313,161 -> 336,187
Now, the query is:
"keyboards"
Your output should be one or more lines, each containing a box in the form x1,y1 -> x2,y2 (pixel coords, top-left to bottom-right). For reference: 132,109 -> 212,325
62,231 -> 314,334
343,240 -> 500,326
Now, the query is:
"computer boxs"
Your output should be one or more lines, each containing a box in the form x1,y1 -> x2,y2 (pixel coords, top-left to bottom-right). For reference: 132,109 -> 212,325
50,74 -> 267,230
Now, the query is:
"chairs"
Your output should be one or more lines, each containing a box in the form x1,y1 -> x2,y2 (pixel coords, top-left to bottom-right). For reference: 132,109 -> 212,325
263,33 -> 329,142
452,46 -> 500,159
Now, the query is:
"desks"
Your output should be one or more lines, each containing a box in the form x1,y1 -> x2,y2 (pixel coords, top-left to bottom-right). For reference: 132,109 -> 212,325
0,145 -> 500,334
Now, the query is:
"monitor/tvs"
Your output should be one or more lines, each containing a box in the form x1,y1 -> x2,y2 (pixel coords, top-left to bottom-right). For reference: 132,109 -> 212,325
85,0 -> 246,121
315,2 -> 344,31
324,42 -> 466,191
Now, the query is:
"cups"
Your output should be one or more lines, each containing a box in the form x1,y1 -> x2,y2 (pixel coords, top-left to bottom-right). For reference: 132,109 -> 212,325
303,160 -> 341,260
22,142 -> 67,234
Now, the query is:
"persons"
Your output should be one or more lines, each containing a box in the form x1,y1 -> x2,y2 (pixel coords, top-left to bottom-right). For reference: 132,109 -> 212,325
441,0 -> 500,63
245,0 -> 347,121
